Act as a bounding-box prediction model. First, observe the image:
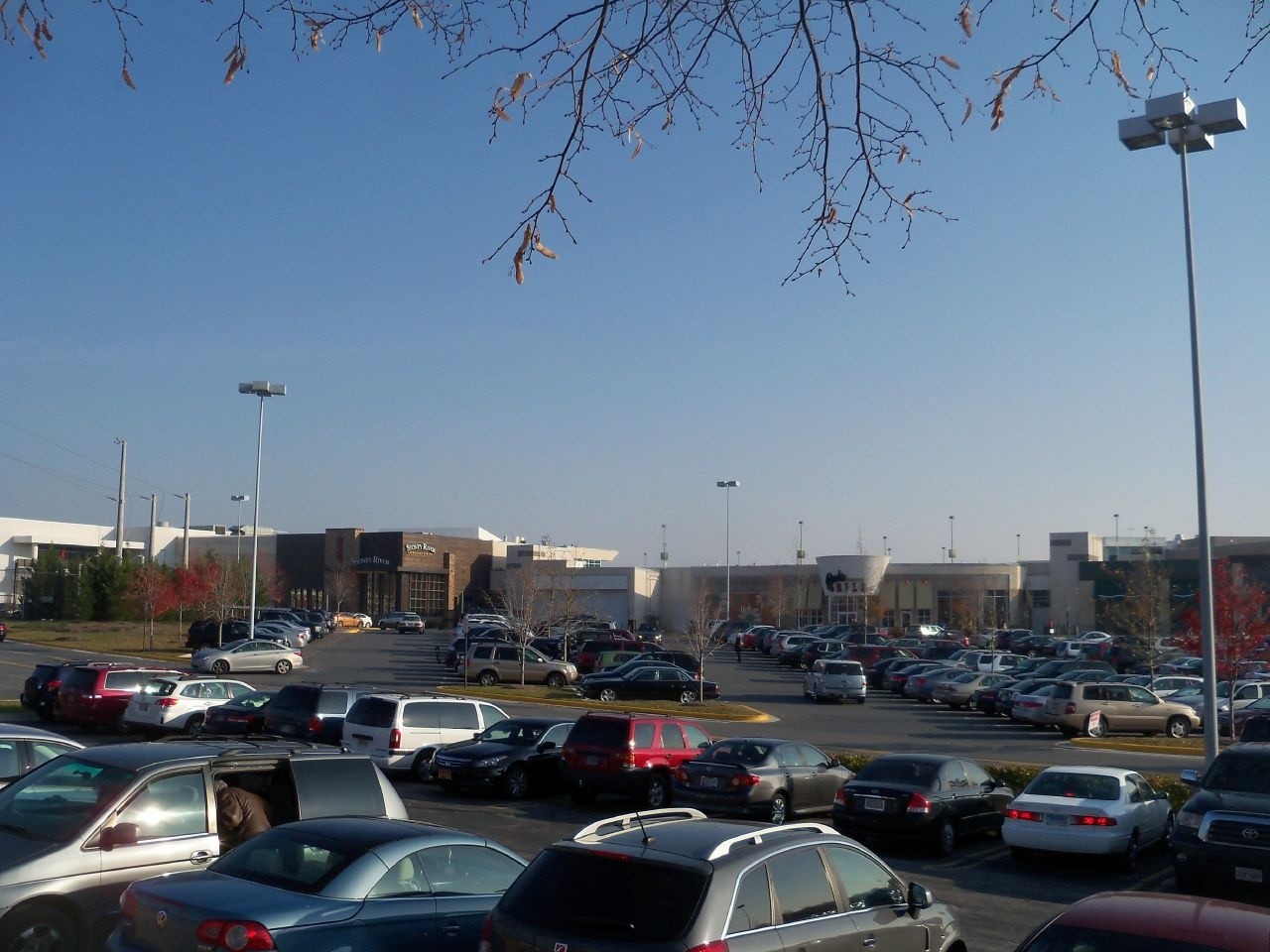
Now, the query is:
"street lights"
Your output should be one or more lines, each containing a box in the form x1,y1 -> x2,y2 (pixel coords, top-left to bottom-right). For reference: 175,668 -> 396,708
942,547 -> 946,563
949,515 -> 955,563
717,480 -> 740,621
238,380 -> 286,639
888,548 -> 891,555
799,521 -> 803,565
1144,526 -> 1148,546
1118,92 -> 1247,774
231,496 -> 249,564
1114,514 -> 1119,561
883,536 -> 886,555
1017,534 -> 1020,561
662,525 -> 667,568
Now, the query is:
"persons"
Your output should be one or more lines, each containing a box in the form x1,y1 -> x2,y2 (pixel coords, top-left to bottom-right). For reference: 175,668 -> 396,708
734,633 -> 741,664
968,613 -> 979,628
609,620 -> 617,630
1048,619 -> 1055,635
214,780 -> 272,853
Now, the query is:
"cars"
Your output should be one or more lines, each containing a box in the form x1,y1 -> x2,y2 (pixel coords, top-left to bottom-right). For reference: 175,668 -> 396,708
705,619 -> 1270,736
0,659 -> 1270,952
446,613 -> 720,703
185,608 -> 426,675
